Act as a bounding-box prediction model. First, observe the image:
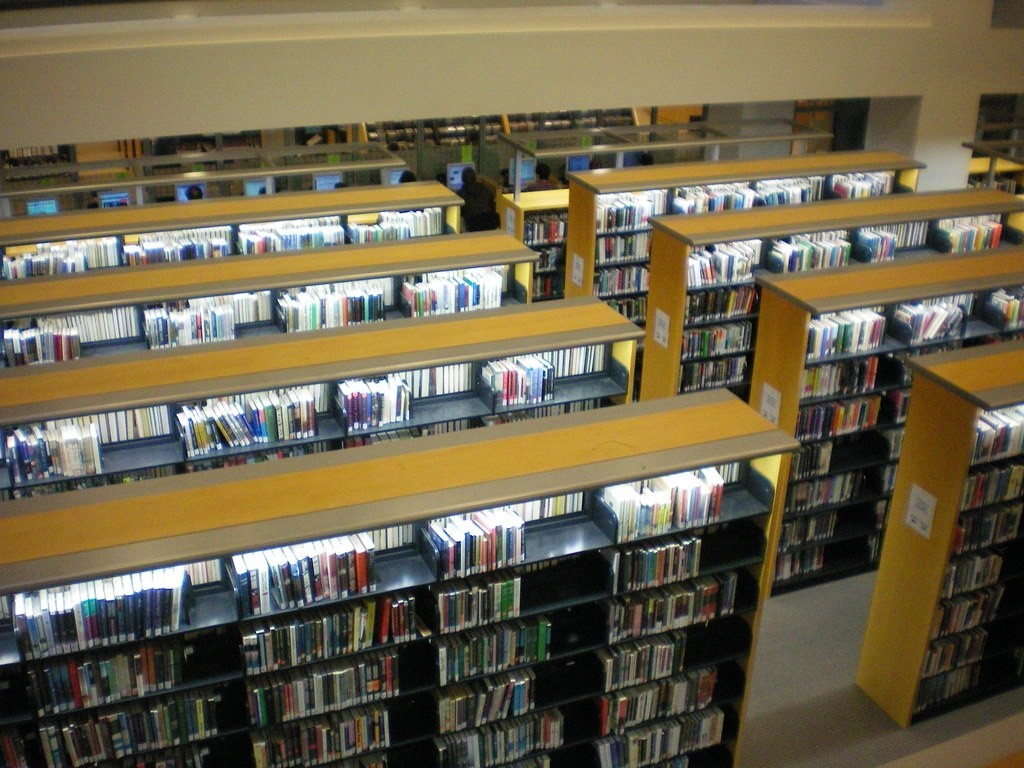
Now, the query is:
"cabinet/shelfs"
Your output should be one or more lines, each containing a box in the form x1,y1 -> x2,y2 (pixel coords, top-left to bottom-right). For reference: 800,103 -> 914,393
0,106 -> 1024,768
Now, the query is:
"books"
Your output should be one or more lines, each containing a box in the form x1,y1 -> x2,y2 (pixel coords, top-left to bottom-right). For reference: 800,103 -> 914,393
25,109 -> 635,189
0,344 -> 608,505
772,282 -> 1024,580
0,458 -> 741,768
593,172 -> 894,325
911,405 -> 1024,716
0,208 -> 510,368
678,213 -> 1003,394
525,210 -> 569,299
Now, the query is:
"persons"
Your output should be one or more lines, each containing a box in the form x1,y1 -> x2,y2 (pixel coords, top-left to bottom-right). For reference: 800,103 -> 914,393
182,164 -> 557,231
